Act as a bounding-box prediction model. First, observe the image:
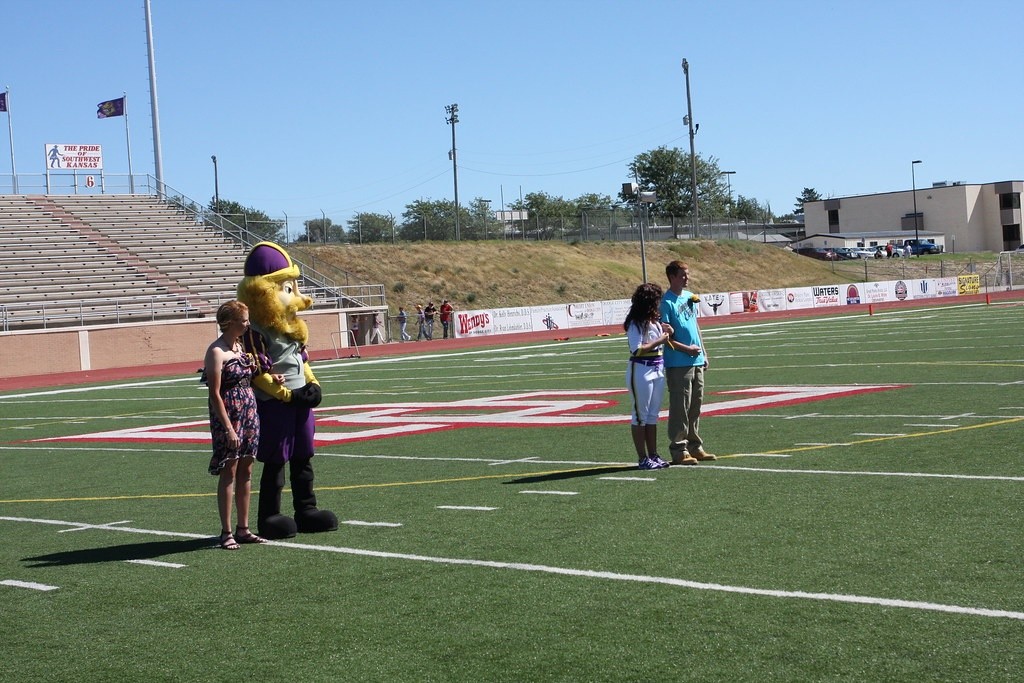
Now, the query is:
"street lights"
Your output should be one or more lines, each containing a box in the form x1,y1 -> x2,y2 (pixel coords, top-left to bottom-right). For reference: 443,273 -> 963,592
211,154 -> 219,213
681,57 -> 700,234
720,170 -> 736,217
445,102 -> 472,248
912,159 -> 923,257
622,161 -> 654,281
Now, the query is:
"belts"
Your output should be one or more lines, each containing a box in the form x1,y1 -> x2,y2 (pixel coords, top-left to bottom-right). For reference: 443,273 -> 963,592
630,358 -> 664,366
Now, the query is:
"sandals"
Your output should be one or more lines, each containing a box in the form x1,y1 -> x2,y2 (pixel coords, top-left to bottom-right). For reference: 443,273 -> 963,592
235,525 -> 268,543
220,529 -> 240,550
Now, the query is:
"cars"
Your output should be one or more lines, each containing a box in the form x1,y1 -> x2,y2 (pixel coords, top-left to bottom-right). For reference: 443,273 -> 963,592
827,245 -> 908,261
792,247 -> 837,262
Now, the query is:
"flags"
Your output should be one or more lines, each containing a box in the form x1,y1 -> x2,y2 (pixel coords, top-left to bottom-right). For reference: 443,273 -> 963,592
97,97 -> 124,118
0,93 -> 7,111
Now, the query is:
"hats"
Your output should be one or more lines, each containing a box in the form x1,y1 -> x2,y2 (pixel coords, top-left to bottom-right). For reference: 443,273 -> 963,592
429,301 -> 434,305
415,305 -> 421,309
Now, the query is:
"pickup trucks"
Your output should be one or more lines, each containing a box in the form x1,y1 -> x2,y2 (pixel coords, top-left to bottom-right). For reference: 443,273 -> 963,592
899,238 -> 942,256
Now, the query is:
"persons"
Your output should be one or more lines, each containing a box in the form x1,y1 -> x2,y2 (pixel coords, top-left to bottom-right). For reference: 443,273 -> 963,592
440,300 -> 454,339
886,243 -> 893,259
624,284 -> 674,469
424,302 -> 437,340
415,305 -> 430,341
371,310 -> 387,343
876,248 -> 883,258
397,307 -> 411,340
351,315 -> 359,346
659,260 -> 717,464
904,242 -> 912,258
204,301 -> 286,550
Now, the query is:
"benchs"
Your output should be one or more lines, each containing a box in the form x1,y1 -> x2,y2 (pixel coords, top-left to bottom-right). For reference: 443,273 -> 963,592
0,194 -> 339,329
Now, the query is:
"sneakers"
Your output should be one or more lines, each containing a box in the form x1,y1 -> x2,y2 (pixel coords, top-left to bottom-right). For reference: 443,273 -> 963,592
639,456 -> 659,469
652,454 -> 669,467
693,451 -> 716,460
674,455 -> 698,464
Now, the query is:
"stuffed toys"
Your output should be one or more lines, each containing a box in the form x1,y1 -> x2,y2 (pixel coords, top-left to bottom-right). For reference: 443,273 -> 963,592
238,242 -> 339,539
